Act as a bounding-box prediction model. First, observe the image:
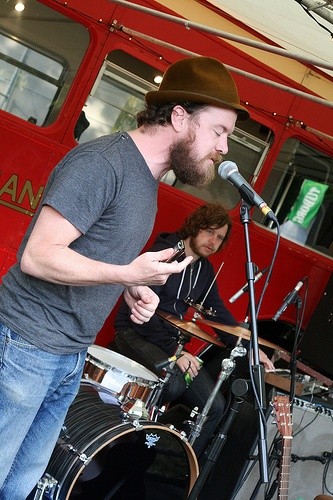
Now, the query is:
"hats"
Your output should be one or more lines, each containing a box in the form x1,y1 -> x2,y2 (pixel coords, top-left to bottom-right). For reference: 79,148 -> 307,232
145,56 -> 251,121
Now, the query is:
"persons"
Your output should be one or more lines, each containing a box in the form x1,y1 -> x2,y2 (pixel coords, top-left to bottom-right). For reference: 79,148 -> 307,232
0,56 -> 250,500
113,203 -> 275,461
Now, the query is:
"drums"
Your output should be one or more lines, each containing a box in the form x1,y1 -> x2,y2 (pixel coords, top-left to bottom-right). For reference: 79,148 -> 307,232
85,343 -> 158,408
28,380 -> 199,500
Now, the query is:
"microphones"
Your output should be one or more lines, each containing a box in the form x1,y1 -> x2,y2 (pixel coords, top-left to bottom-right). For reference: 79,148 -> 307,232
218,161 -> 279,222
229,267 -> 267,303
273,281 -> 303,322
151,354 -> 184,372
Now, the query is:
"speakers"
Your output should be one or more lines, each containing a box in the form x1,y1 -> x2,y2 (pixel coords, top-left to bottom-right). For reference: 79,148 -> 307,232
205,393 -> 333,500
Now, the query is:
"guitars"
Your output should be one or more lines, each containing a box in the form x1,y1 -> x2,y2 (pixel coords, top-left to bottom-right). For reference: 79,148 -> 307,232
268,396 -> 295,500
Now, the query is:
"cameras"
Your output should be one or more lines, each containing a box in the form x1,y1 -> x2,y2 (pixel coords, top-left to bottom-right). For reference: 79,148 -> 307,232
164,240 -> 185,264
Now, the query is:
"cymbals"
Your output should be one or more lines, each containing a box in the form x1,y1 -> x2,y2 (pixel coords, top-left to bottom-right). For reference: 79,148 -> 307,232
199,320 -> 286,354
152,307 -> 227,349
